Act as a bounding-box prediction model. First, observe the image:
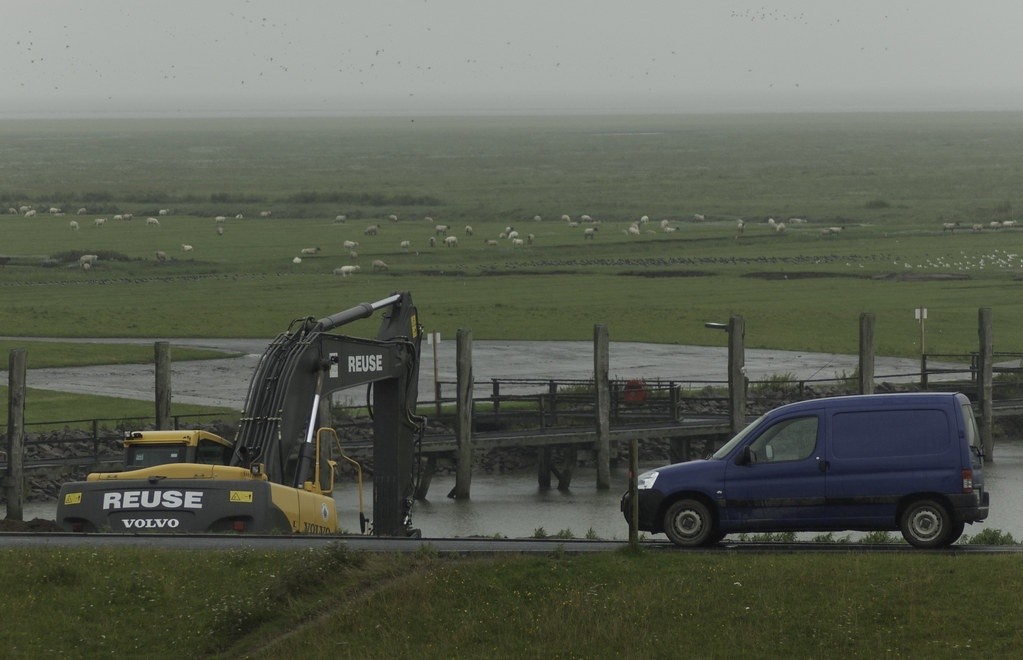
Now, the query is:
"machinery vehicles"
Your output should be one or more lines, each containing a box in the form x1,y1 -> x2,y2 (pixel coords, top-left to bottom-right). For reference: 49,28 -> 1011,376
54,289 -> 430,537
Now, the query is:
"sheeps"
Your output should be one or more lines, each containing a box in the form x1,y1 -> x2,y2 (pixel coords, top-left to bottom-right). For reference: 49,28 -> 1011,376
463,224 -> 472,236
1002,220 -> 1017,230
990,221 -> 1001,232
789,218 -> 809,224
561,214 -> 599,240
942,222 -> 960,231
76,243 -> 194,271
484,225 -> 534,249
215,211 -> 272,236
736,219 -> 745,233
291,212 -> 458,277
8,202 -> 170,230
533,215 -> 541,224
972,224 -> 984,232
844,247 -> 1023,270
621,212 -> 707,236
820,225 -> 845,239
768,218 -> 785,233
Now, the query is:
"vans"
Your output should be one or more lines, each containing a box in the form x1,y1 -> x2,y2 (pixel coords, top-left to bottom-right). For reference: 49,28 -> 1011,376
613,391 -> 990,550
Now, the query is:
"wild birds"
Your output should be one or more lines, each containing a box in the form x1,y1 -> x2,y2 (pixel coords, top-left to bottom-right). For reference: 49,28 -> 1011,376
8,1 -> 899,128
